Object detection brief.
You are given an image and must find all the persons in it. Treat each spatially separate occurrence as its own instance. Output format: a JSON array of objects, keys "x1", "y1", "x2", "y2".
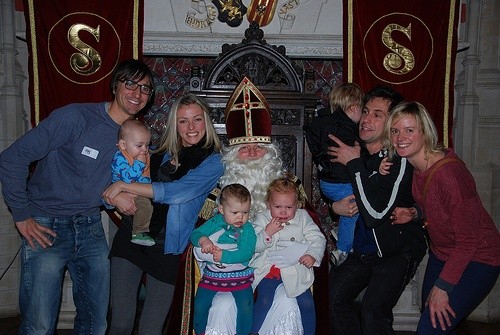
[
  {"x1": 101, "y1": 92, "x2": 226, "y2": 334},
  {"x1": 192, "y1": 125, "x2": 328, "y2": 335},
  {"x1": 384, "y1": 99, "x2": 500, "y2": 335},
  {"x1": 0, "y1": 59, "x2": 156, "y2": 335},
  {"x1": 327, "y1": 86, "x2": 430, "y2": 335},
  {"x1": 303, "y1": 83, "x2": 395, "y2": 267}
]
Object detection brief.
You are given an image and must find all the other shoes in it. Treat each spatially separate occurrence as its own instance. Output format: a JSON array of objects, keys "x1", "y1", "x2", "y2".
[{"x1": 131, "y1": 233, "x2": 156, "y2": 246}]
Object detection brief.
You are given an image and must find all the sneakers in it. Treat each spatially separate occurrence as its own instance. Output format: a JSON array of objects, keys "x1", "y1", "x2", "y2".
[
  {"x1": 331, "y1": 227, "x2": 353, "y2": 252},
  {"x1": 330, "y1": 249, "x2": 348, "y2": 267}
]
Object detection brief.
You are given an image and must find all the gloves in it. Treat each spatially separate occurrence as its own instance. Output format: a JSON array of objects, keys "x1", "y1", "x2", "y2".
[
  {"x1": 265, "y1": 240, "x2": 309, "y2": 269},
  {"x1": 195, "y1": 229, "x2": 239, "y2": 268}
]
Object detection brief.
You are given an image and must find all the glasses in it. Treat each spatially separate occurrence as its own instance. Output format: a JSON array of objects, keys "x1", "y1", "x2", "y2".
[
  {"x1": 118, "y1": 79, "x2": 152, "y2": 95},
  {"x1": 240, "y1": 145, "x2": 264, "y2": 152}
]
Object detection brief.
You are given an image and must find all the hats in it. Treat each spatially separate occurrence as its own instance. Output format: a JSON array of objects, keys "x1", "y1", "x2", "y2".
[{"x1": 224, "y1": 76, "x2": 272, "y2": 146}]
